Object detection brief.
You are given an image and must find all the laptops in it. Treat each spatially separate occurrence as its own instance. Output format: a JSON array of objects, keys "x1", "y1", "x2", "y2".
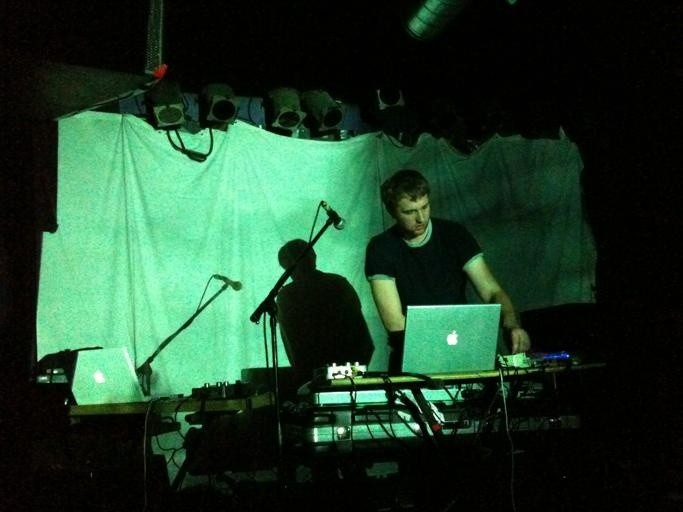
[
  {"x1": 58, "y1": 346, "x2": 156, "y2": 407},
  {"x1": 398, "y1": 302, "x2": 503, "y2": 375}
]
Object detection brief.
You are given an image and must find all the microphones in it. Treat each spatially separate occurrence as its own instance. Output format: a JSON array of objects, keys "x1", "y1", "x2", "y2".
[{"x1": 320, "y1": 199, "x2": 346, "y2": 231}]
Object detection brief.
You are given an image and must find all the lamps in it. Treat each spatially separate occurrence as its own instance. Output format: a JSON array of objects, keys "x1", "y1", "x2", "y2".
[
  {"x1": 301, "y1": 86, "x2": 347, "y2": 134},
  {"x1": 268, "y1": 85, "x2": 307, "y2": 134},
  {"x1": 149, "y1": 79, "x2": 186, "y2": 131},
  {"x1": 200, "y1": 79, "x2": 244, "y2": 125}
]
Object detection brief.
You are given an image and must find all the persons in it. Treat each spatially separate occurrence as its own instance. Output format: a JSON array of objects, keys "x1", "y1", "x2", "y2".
[
  {"x1": 365, "y1": 169, "x2": 531, "y2": 375},
  {"x1": 275, "y1": 239, "x2": 375, "y2": 367}
]
